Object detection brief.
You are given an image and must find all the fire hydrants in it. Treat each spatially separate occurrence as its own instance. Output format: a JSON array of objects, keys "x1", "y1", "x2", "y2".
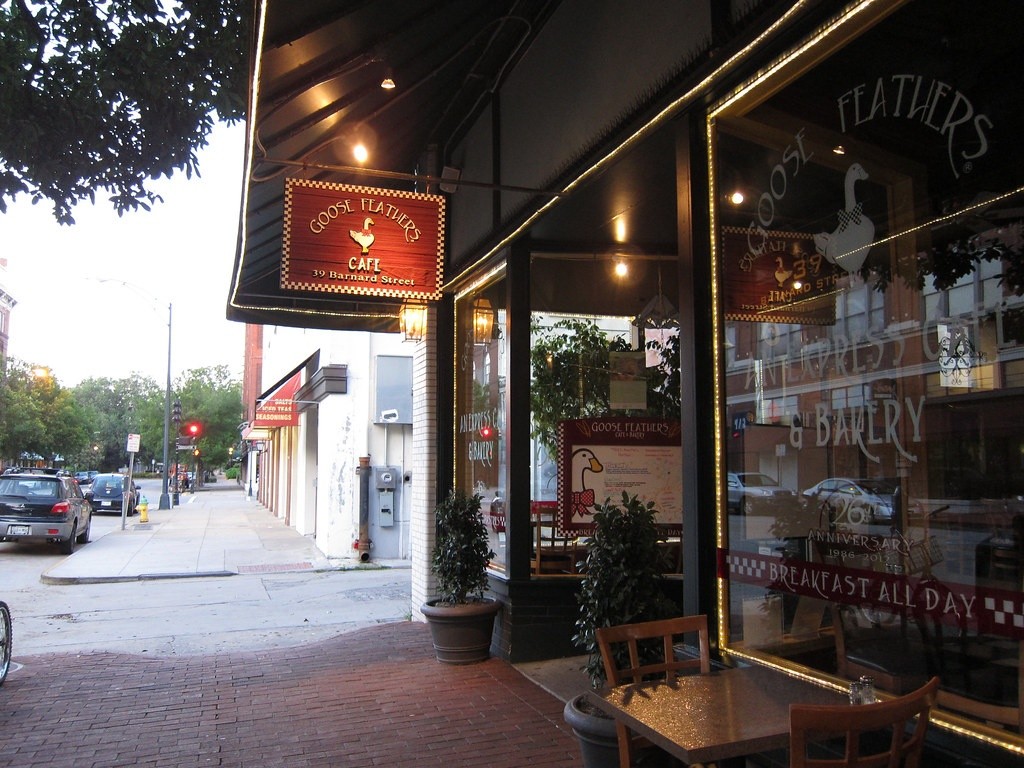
[{"x1": 136, "y1": 495, "x2": 150, "y2": 522}]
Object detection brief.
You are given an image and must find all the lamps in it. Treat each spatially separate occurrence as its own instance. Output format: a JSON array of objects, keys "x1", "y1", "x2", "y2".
[
  {"x1": 472, "y1": 293, "x2": 495, "y2": 347},
  {"x1": 631, "y1": 265, "x2": 679, "y2": 330},
  {"x1": 256, "y1": 440, "x2": 268, "y2": 457},
  {"x1": 398, "y1": 298, "x2": 428, "y2": 343}
]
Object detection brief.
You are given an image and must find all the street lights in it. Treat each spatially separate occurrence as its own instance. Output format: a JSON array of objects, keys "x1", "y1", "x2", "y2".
[
  {"x1": 99, "y1": 277, "x2": 172, "y2": 510},
  {"x1": 32, "y1": 367, "x2": 48, "y2": 378}
]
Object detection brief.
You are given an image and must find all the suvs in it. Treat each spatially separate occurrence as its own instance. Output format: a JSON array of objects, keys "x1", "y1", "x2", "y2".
[
  {"x1": 0, "y1": 466, "x2": 95, "y2": 555},
  {"x1": 727, "y1": 471, "x2": 796, "y2": 516},
  {"x1": 170, "y1": 472, "x2": 190, "y2": 490}
]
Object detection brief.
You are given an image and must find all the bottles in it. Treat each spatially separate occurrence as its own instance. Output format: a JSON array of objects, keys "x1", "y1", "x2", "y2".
[{"x1": 850, "y1": 675, "x2": 876, "y2": 705}]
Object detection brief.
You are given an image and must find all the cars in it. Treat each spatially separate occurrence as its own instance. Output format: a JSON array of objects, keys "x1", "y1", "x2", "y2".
[
  {"x1": 74, "y1": 471, "x2": 92, "y2": 484},
  {"x1": 90, "y1": 470, "x2": 100, "y2": 479},
  {"x1": 186, "y1": 472, "x2": 197, "y2": 483},
  {"x1": 84, "y1": 473, "x2": 141, "y2": 517},
  {"x1": 803, "y1": 477, "x2": 924, "y2": 527},
  {"x1": 3, "y1": 467, "x2": 72, "y2": 477}
]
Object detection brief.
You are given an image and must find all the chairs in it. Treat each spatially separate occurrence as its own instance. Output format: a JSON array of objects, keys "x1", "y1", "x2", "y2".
[
  {"x1": 594, "y1": 614, "x2": 747, "y2": 768},
  {"x1": 789, "y1": 675, "x2": 938, "y2": 767},
  {"x1": 530, "y1": 499, "x2": 578, "y2": 577}
]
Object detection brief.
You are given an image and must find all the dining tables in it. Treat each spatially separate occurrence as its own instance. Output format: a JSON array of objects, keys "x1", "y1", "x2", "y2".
[{"x1": 588, "y1": 666, "x2": 852, "y2": 768}]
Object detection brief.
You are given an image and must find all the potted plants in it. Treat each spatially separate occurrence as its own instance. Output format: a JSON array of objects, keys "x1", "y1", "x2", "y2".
[
  {"x1": 421, "y1": 485, "x2": 502, "y2": 665},
  {"x1": 562, "y1": 486, "x2": 683, "y2": 768}
]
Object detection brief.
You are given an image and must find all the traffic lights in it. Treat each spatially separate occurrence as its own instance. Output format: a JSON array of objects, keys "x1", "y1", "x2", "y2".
[{"x1": 190, "y1": 422, "x2": 200, "y2": 435}]
